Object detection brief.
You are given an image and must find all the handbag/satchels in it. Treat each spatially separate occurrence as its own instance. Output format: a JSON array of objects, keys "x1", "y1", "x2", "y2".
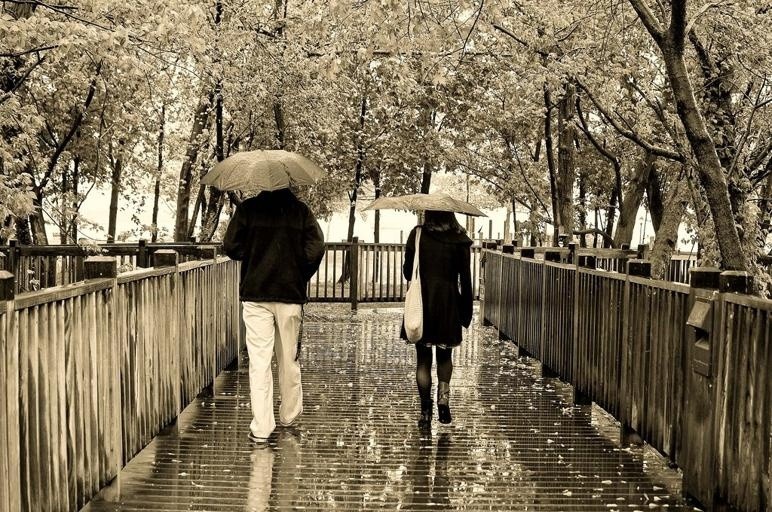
[{"x1": 402, "y1": 226, "x2": 427, "y2": 345}]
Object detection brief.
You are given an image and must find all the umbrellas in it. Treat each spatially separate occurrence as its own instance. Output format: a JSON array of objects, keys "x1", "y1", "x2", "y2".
[
  {"x1": 198, "y1": 147, "x2": 328, "y2": 192},
  {"x1": 361, "y1": 190, "x2": 488, "y2": 218}
]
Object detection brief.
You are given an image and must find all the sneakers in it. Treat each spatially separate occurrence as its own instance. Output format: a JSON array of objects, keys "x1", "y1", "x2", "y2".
[
  {"x1": 247, "y1": 430, "x2": 268, "y2": 444},
  {"x1": 279, "y1": 405, "x2": 304, "y2": 427}
]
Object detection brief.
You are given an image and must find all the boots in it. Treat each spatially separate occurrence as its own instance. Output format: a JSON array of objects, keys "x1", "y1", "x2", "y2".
[
  {"x1": 417, "y1": 398, "x2": 436, "y2": 430},
  {"x1": 438, "y1": 381, "x2": 452, "y2": 424}
]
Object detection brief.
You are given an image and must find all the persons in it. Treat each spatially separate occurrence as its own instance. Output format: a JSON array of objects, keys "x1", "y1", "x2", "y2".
[
  {"x1": 223, "y1": 187, "x2": 326, "y2": 445},
  {"x1": 244, "y1": 428, "x2": 301, "y2": 512},
  {"x1": 401, "y1": 421, "x2": 457, "y2": 511},
  {"x1": 400, "y1": 210, "x2": 473, "y2": 427}
]
